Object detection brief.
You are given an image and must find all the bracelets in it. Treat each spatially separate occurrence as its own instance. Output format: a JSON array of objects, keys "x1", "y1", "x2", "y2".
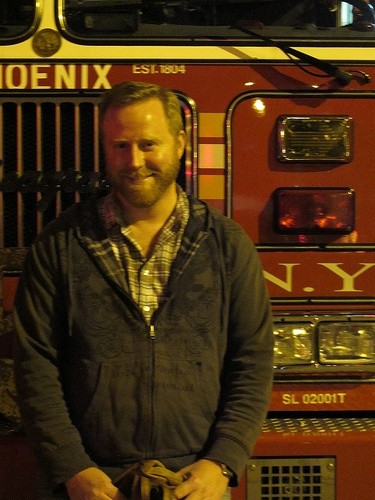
[{"x1": 204, "y1": 448, "x2": 241, "y2": 486}]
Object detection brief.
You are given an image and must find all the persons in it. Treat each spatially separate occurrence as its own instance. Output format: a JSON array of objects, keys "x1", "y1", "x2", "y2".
[{"x1": 11, "y1": 81, "x2": 275, "y2": 500}]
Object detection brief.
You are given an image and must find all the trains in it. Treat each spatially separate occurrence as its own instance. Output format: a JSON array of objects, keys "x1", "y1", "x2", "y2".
[{"x1": 0, "y1": 0, "x2": 375, "y2": 500}]
